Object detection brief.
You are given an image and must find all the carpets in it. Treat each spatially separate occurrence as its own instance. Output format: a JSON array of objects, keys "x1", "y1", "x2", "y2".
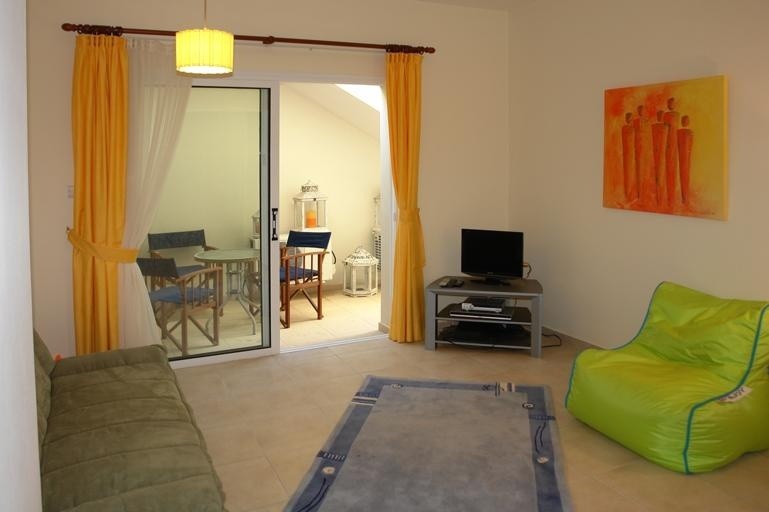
[{"x1": 277, "y1": 372, "x2": 576, "y2": 511}]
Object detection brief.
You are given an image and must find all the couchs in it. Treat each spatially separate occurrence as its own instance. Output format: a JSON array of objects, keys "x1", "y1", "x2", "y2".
[{"x1": 34, "y1": 330, "x2": 225, "y2": 511}]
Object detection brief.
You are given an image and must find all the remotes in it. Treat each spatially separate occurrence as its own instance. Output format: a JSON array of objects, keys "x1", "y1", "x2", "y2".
[
  {"x1": 455, "y1": 280, "x2": 464, "y2": 287},
  {"x1": 447, "y1": 279, "x2": 456, "y2": 288},
  {"x1": 439, "y1": 277, "x2": 450, "y2": 287}
]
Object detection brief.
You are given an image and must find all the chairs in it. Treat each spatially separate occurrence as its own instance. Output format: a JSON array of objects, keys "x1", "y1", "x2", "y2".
[
  {"x1": 132, "y1": 253, "x2": 225, "y2": 358},
  {"x1": 562, "y1": 277, "x2": 768, "y2": 478},
  {"x1": 247, "y1": 230, "x2": 334, "y2": 328},
  {"x1": 146, "y1": 228, "x2": 226, "y2": 316}
]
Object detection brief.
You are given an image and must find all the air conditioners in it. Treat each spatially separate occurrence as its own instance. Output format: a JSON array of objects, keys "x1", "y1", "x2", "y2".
[
  {"x1": 369, "y1": 226, "x2": 383, "y2": 282},
  {"x1": 250, "y1": 228, "x2": 334, "y2": 286}
]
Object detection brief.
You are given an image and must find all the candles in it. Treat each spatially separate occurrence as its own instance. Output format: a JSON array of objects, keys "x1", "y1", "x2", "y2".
[{"x1": 306, "y1": 210, "x2": 317, "y2": 229}]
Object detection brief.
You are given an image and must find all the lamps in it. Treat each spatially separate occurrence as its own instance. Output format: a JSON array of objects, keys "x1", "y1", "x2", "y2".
[{"x1": 171, "y1": 1, "x2": 236, "y2": 77}]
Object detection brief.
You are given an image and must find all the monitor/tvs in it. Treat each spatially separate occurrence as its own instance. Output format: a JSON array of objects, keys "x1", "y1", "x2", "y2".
[{"x1": 460, "y1": 228, "x2": 524, "y2": 285}]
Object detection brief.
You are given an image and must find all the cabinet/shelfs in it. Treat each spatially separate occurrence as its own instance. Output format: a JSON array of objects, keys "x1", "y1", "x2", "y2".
[{"x1": 423, "y1": 274, "x2": 546, "y2": 361}]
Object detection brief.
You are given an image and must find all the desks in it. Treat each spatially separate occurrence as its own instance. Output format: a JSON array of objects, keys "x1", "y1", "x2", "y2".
[{"x1": 192, "y1": 246, "x2": 258, "y2": 338}]
